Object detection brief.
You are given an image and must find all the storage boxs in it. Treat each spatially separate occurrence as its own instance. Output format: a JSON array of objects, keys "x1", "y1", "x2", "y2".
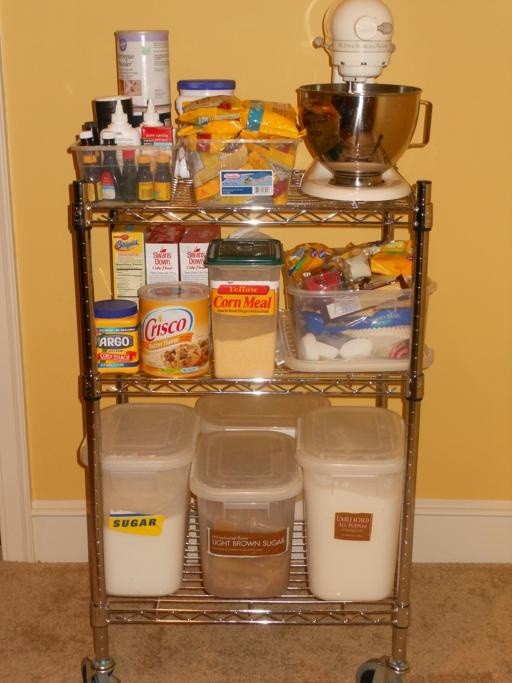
[
  {"x1": 205, "y1": 237, "x2": 438, "y2": 379},
  {"x1": 69, "y1": 140, "x2": 301, "y2": 208}
]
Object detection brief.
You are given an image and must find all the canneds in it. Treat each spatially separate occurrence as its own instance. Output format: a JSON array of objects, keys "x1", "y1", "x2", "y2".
[{"x1": 97, "y1": 299, "x2": 139, "y2": 374}]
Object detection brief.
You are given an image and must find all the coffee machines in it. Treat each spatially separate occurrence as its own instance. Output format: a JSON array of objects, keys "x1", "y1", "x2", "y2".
[{"x1": 294, "y1": 0, "x2": 433, "y2": 202}]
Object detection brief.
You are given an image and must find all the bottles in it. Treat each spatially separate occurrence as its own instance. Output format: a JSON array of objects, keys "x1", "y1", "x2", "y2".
[{"x1": 77, "y1": 96, "x2": 172, "y2": 204}]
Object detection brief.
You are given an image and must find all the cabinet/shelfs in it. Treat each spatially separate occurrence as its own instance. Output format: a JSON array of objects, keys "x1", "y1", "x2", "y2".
[{"x1": 73, "y1": 179, "x2": 432, "y2": 683}]
